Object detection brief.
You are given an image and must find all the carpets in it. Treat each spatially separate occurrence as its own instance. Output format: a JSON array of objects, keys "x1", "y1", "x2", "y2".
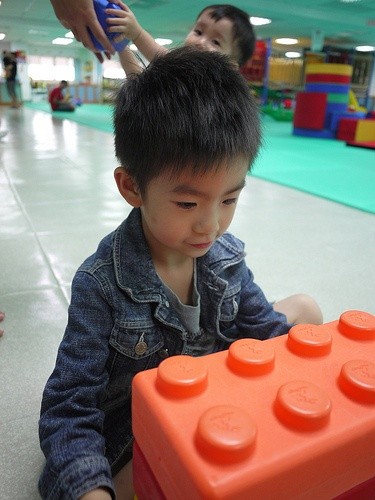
[{"x1": 23, "y1": 97, "x2": 375, "y2": 219}]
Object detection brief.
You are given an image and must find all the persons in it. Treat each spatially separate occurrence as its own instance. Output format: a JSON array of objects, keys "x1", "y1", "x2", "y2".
[
  {"x1": 2, "y1": 51, "x2": 20, "y2": 109},
  {"x1": 50, "y1": 0, "x2": 117, "y2": 62},
  {"x1": 103, "y1": 0, "x2": 256, "y2": 83},
  {"x1": 48, "y1": 79, "x2": 75, "y2": 111},
  {"x1": 33, "y1": 44, "x2": 322, "y2": 500}
]
